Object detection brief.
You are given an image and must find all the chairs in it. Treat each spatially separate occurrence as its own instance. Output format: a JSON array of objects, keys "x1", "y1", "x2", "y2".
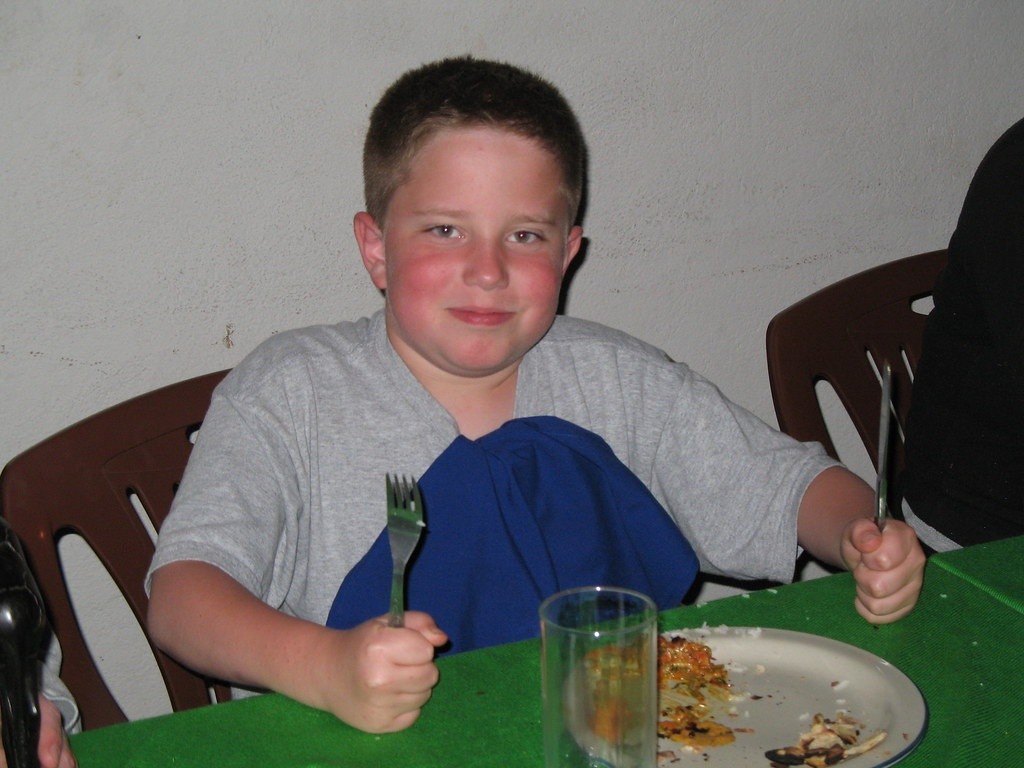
[
  {"x1": 767, "y1": 249, "x2": 969, "y2": 582},
  {"x1": 0, "y1": 368, "x2": 232, "y2": 731}
]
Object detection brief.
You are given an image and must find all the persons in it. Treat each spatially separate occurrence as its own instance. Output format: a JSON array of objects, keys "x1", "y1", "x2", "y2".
[
  {"x1": 900, "y1": 117, "x2": 1024, "y2": 559},
  {"x1": 0, "y1": 518, "x2": 82, "y2": 768},
  {"x1": 144, "y1": 55, "x2": 925, "y2": 735}
]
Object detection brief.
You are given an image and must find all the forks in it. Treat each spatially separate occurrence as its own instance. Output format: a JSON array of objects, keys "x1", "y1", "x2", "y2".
[{"x1": 385, "y1": 472, "x2": 423, "y2": 626}]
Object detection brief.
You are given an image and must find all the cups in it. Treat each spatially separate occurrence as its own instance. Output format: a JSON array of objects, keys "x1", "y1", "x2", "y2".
[{"x1": 538, "y1": 586, "x2": 659, "y2": 768}]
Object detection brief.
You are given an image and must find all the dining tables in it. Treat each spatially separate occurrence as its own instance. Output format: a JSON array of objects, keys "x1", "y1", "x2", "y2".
[{"x1": 71, "y1": 534, "x2": 1024, "y2": 768}]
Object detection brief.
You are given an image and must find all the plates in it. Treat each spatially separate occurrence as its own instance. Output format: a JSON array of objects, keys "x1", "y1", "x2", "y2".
[{"x1": 562, "y1": 626, "x2": 930, "y2": 768}]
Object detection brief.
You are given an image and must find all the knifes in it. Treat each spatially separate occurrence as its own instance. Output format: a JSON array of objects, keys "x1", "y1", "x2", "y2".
[{"x1": 873, "y1": 360, "x2": 892, "y2": 631}]
